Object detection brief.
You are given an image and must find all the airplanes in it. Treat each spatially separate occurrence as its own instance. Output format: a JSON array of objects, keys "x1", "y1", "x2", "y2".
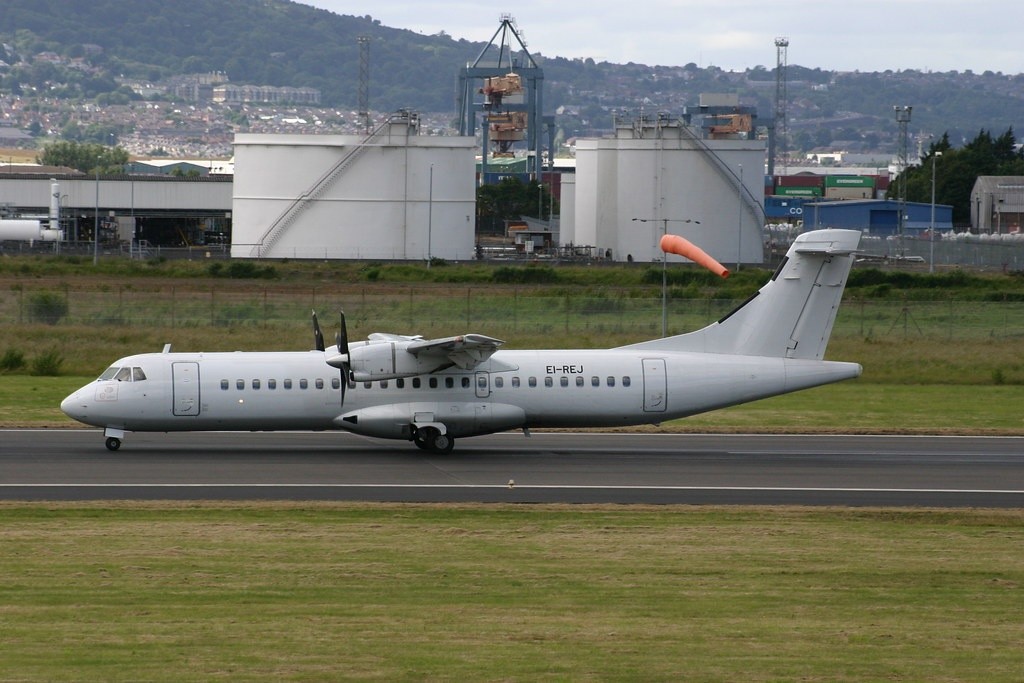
[{"x1": 60, "y1": 225, "x2": 864, "y2": 455}]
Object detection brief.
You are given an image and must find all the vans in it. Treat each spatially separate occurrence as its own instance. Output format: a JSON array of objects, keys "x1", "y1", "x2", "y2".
[{"x1": 203, "y1": 230, "x2": 231, "y2": 251}]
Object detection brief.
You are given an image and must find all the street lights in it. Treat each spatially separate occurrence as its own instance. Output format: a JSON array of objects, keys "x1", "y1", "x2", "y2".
[
  {"x1": 924, "y1": 150, "x2": 943, "y2": 274},
  {"x1": 94, "y1": 155, "x2": 103, "y2": 266},
  {"x1": 738, "y1": 161, "x2": 744, "y2": 279},
  {"x1": 127, "y1": 160, "x2": 135, "y2": 261},
  {"x1": 547, "y1": 160, "x2": 555, "y2": 231},
  {"x1": 537, "y1": 183, "x2": 542, "y2": 221},
  {"x1": 998, "y1": 198, "x2": 1004, "y2": 237}
]
